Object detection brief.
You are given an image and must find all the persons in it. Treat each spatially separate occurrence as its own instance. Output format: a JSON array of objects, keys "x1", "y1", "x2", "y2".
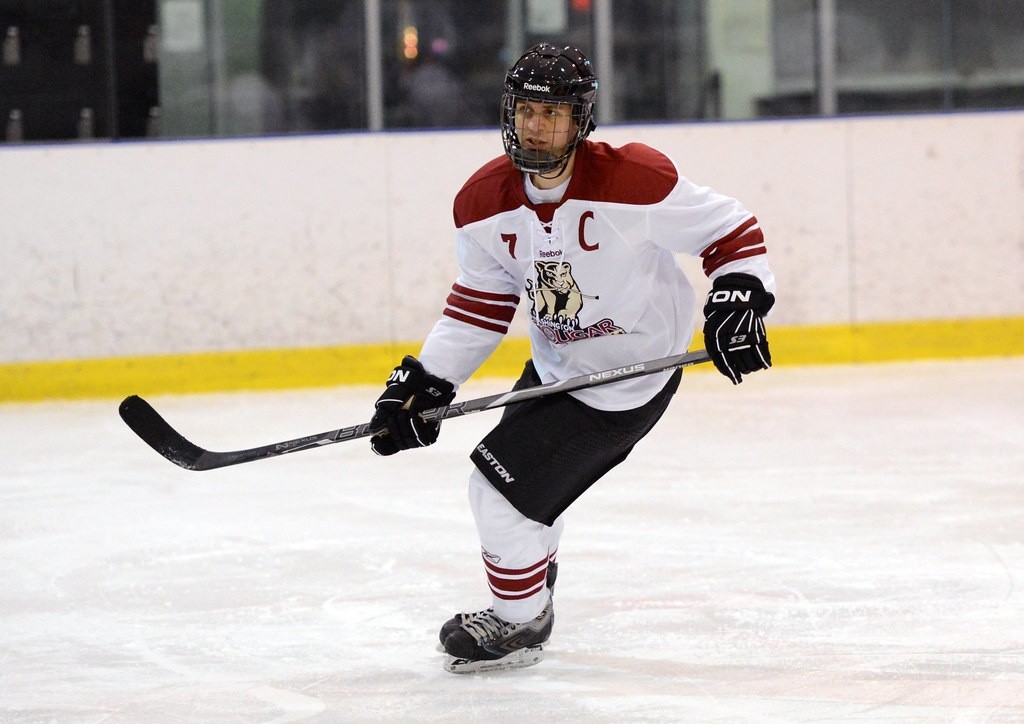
[
  {"x1": 370, "y1": 42, "x2": 776, "y2": 673},
  {"x1": 228, "y1": 31, "x2": 355, "y2": 135}
]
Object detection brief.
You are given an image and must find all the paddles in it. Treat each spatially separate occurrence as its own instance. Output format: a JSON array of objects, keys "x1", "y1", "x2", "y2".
[{"x1": 116, "y1": 349, "x2": 716, "y2": 472}]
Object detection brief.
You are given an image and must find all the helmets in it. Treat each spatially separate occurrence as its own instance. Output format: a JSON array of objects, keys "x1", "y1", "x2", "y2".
[{"x1": 500, "y1": 42, "x2": 599, "y2": 176}]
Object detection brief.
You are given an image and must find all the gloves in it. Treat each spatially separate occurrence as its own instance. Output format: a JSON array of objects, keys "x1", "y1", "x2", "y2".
[
  {"x1": 370, "y1": 356, "x2": 457, "y2": 456},
  {"x1": 703, "y1": 273, "x2": 774, "y2": 385}
]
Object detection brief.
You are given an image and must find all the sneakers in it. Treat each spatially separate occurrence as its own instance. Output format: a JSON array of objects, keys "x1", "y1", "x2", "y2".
[
  {"x1": 440, "y1": 561, "x2": 558, "y2": 640},
  {"x1": 444, "y1": 594, "x2": 555, "y2": 672}
]
